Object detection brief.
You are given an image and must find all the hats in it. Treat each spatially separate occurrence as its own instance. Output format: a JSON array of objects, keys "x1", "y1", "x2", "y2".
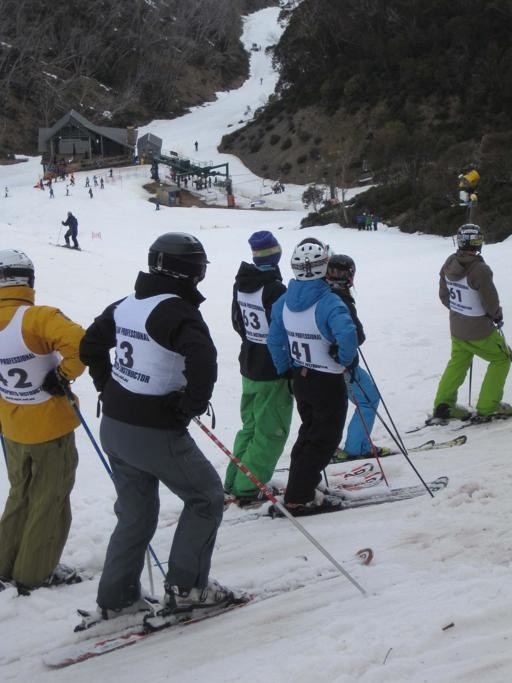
[{"x1": 248, "y1": 229, "x2": 282, "y2": 267}]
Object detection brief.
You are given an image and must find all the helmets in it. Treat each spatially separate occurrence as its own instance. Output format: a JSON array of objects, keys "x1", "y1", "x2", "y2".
[
  {"x1": 147, "y1": 230, "x2": 211, "y2": 286},
  {"x1": 0, "y1": 248, "x2": 35, "y2": 290},
  {"x1": 325, "y1": 253, "x2": 357, "y2": 288},
  {"x1": 290, "y1": 236, "x2": 331, "y2": 282},
  {"x1": 455, "y1": 222, "x2": 484, "y2": 253}
]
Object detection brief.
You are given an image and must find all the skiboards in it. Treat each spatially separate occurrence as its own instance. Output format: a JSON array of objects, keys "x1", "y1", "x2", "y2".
[
  {"x1": 221, "y1": 437, "x2": 467, "y2": 518},
  {"x1": 41, "y1": 549, "x2": 374, "y2": 670},
  {"x1": 405, "y1": 412, "x2": 509, "y2": 432}
]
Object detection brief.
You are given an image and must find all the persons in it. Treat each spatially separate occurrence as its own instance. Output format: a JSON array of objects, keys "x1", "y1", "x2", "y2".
[
  {"x1": 194, "y1": 139, "x2": 199, "y2": 151},
  {"x1": 223, "y1": 229, "x2": 297, "y2": 508},
  {"x1": 75, "y1": 230, "x2": 239, "y2": 623},
  {"x1": 434, "y1": 221, "x2": 511, "y2": 418},
  {"x1": 355, "y1": 211, "x2": 379, "y2": 230},
  {"x1": 155, "y1": 193, "x2": 161, "y2": 211},
  {"x1": 263, "y1": 238, "x2": 359, "y2": 519},
  {"x1": 5, "y1": 186, "x2": 10, "y2": 196},
  {"x1": 322, "y1": 253, "x2": 391, "y2": 459},
  {"x1": 1, "y1": 245, "x2": 90, "y2": 597},
  {"x1": 169, "y1": 163, "x2": 219, "y2": 189},
  {"x1": 61, "y1": 212, "x2": 81, "y2": 248},
  {"x1": 271, "y1": 181, "x2": 285, "y2": 193},
  {"x1": 36, "y1": 157, "x2": 116, "y2": 198}
]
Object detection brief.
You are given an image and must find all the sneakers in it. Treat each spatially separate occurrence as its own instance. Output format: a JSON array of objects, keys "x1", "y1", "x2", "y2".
[
  {"x1": 15, "y1": 563, "x2": 83, "y2": 592},
  {"x1": 223, "y1": 483, "x2": 281, "y2": 506},
  {"x1": 433, "y1": 402, "x2": 512, "y2": 421},
  {"x1": 343, "y1": 444, "x2": 384, "y2": 459},
  {"x1": 62, "y1": 242, "x2": 79, "y2": 249},
  {"x1": 162, "y1": 579, "x2": 236, "y2": 616},
  {"x1": 282, "y1": 485, "x2": 352, "y2": 514},
  {"x1": 94, "y1": 590, "x2": 162, "y2": 621}
]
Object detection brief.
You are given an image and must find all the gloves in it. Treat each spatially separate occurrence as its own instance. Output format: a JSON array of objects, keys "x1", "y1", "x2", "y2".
[
  {"x1": 491, "y1": 306, "x2": 504, "y2": 325},
  {"x1": 281, "y1": 366, "x2": 295, "y2": 381},
  {"x1": 329, "y1": 342, "x2": 360, "y2": 370},
  {"x1": 172, "y1": 385, "x2": 210, "y2": 427},
  {"x1": 41, "y1": 366, "x2": 70, "y2": 399}
]
